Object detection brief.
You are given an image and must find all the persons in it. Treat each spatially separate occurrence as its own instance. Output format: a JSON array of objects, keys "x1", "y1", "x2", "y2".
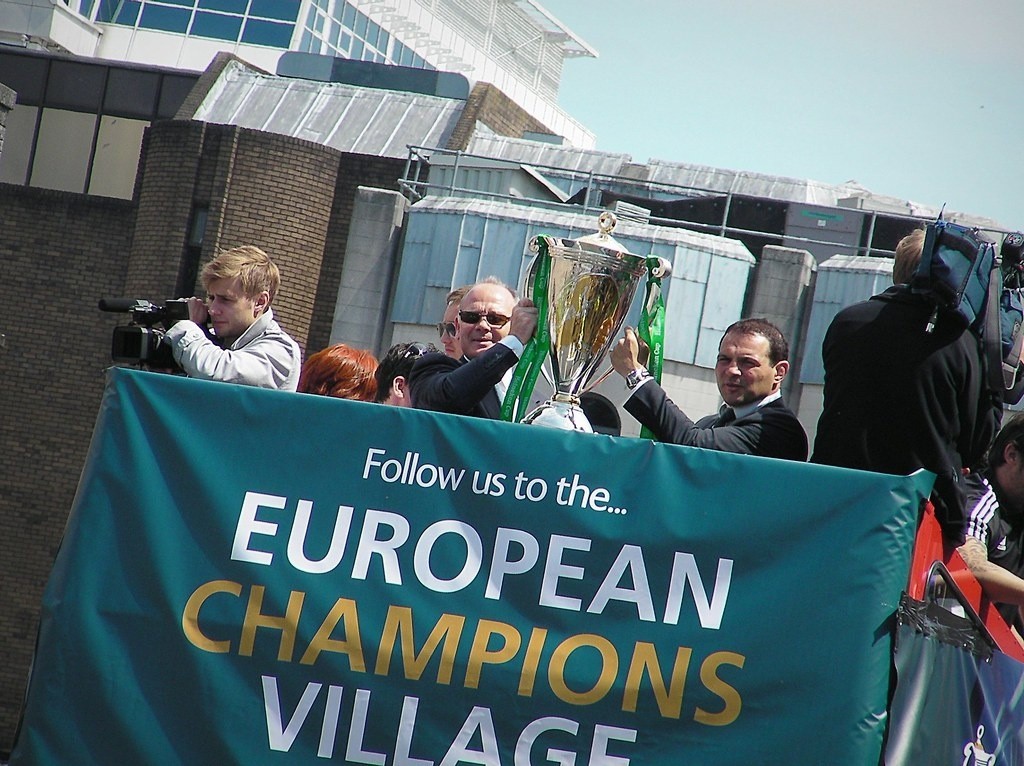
[
  {"x1": 437, "y1": 284, "x2": 474, "y2": 361},
  {"x1": 408, "y1": 276, "x2": 539, "y2": 420},
  {"x1": 811, "y1": 227, "x2": 1024, "y2": 640},
  {"x1": 375, "y1": 340, "x2": 443, "y2": 407},
  {"x1": 608, "y1": 317, "x2": 809, "y2": 462},
  {"x1": 163, "y1": 245, "x2": 302, "y2": 393},
  {"x1": 297, "y1": 344, "x2": 380, "y2": 404}
]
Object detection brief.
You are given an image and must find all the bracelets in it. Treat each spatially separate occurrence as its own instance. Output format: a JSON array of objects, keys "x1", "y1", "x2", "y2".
[{"x1": 626, "y1": 368, "x2": 650, "y2": 389}]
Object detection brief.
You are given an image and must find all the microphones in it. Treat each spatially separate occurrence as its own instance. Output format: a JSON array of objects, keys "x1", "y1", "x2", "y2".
[{"x1": 98, "y1": 299, "x2": 155, "y2": 313}]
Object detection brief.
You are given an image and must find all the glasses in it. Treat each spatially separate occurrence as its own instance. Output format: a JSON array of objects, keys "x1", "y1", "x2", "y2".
[
  {"x1": 388, "y1": 342, "x2": 428, "y2": 383},
  {"x1": 459, "y1": 311, "x2": 511, "y2": 329},
  {"x1": 436, "y1": 323, "x2": 457, "y2": 338}
]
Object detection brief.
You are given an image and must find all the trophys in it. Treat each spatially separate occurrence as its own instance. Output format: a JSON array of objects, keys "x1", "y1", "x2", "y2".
[{"x1": 517, "y1": 212, "x2": 672, "y2": 431}]
